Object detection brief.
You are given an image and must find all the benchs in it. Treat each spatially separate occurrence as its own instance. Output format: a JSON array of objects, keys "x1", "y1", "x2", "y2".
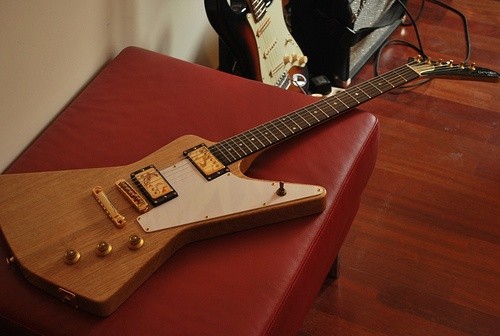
[{"x1": 1, "y1": 43, "x2": 380, "y2": 335}]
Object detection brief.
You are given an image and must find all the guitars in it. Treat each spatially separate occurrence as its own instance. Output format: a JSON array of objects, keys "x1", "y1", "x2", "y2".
[
  {"x1": 0, "y1": 55, "x2": 499, "y2": 317},
  {"x1": 204, "y1": 0, "x2": 308, "y2": 93}
]
